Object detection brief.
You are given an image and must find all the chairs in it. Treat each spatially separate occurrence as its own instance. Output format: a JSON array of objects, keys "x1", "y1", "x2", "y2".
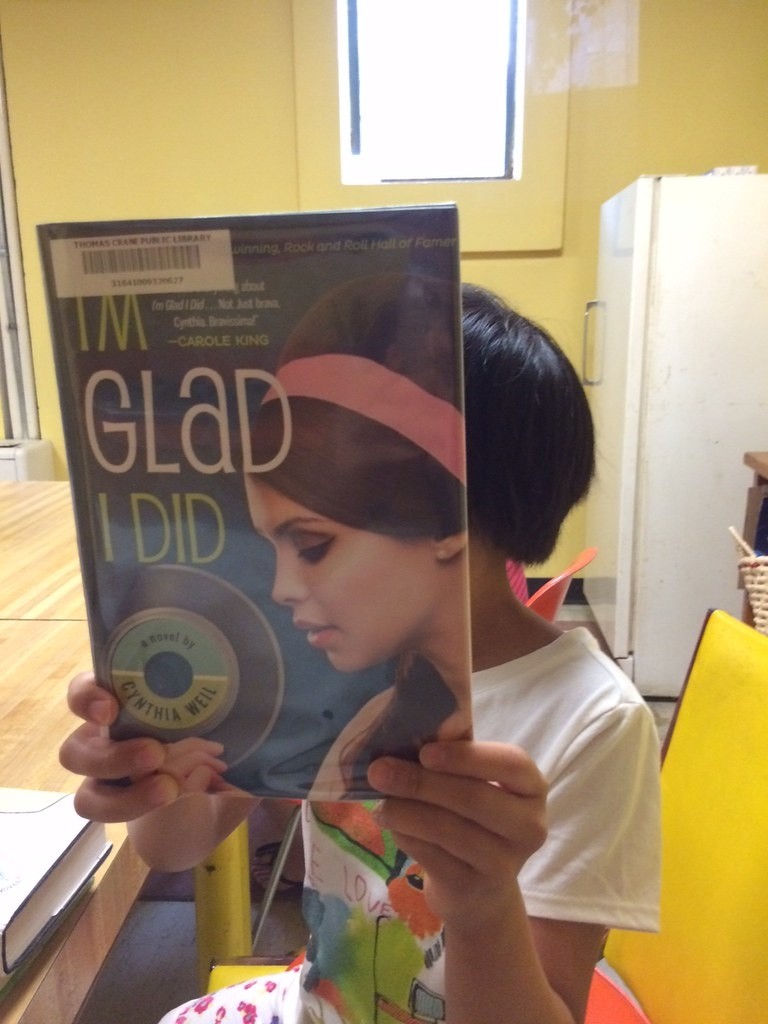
[{"x1": 206, "y1": 608, "x2": 768, "y2": 1024}]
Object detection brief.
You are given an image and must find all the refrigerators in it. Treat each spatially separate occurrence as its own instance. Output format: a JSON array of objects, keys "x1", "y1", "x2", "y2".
[{"x1": 581, "y1": 174, "x2": 768, "y2": 699}]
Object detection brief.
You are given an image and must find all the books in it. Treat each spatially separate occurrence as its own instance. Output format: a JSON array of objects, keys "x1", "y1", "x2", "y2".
[{"x1": 34, "y1": 204, "x2": 476, "y2": 798}]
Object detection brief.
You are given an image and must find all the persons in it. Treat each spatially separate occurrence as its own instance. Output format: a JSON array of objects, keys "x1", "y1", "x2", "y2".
[
  {"x1": 242, "y1": 269, "x2": 474, "y2": 799},
  {"x1": 59, "y1": 282, "x2": 663, "y2": 1024}
]
